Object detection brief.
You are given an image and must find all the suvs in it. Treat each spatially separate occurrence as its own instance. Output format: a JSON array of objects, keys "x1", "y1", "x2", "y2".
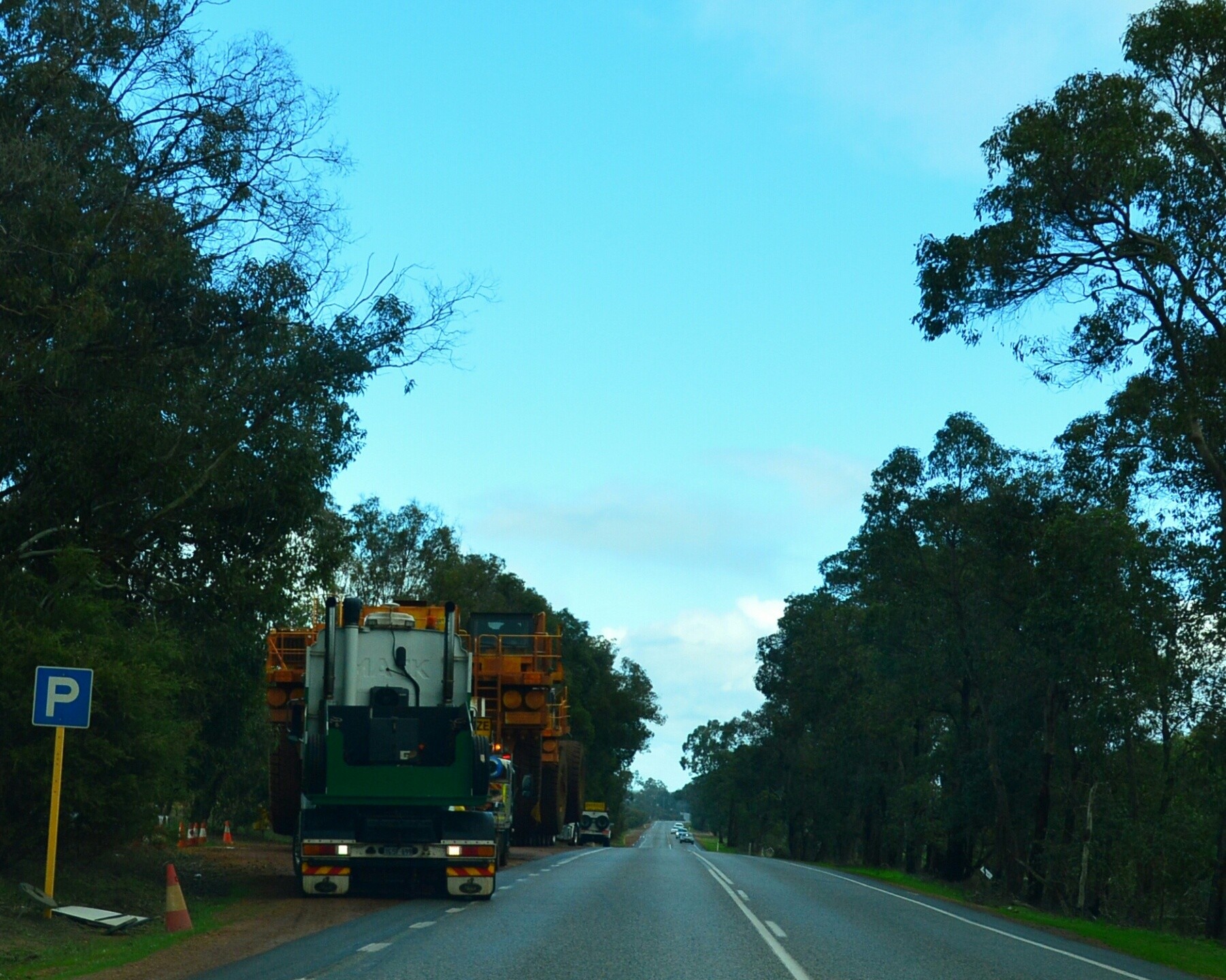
[{"x1": 576, "y1": 811, "x2": 615, "y2": 847}]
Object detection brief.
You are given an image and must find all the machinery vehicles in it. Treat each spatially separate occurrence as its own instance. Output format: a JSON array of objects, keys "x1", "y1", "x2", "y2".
[{"x1": 261, "y1": 590, "x2": 589, "y2": 903}]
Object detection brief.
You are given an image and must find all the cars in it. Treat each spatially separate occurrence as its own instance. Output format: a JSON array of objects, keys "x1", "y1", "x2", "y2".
[{"x1": 670, "y1": 822, "x2": 695, "y2": 845}]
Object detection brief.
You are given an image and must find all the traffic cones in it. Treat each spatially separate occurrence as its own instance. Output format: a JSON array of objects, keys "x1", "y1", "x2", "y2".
[
  {"x1": 197, "y1": 822, "x2": 208, "y2": 845},
  {"x1": 221, "y1": 820, "x2": 234, "y2": 847},
  {"x1": 192, "y1": 821, "x2": 199, "y2": 845},
  {"x1": 185, "y1": 822, "x2": 196, "y2": 846},
  {"x1": 163, "y1": 863, "x2": 193, "y2": 933},
  {"x1": 175, "y1": 821, "x2": 187, "y2": 848}
]
